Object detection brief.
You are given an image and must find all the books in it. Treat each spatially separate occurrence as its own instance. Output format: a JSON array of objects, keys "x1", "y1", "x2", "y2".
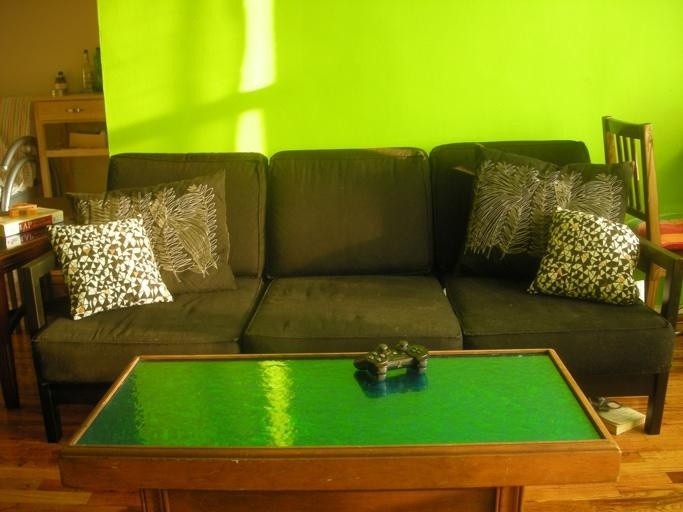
[
  {"x1": 0, "y1": 203, "x2": 64, "y2": 250},
  {"x1": 588, "y1": 396, "x2": 646, "y2": 436}
]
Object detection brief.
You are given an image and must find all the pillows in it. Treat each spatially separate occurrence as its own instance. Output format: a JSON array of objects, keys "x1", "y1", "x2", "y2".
[
  {"x1": 524, "y1": 204, "x2": 642, "y2": 306},
  {"x1": 40, "y1": 214, "x2": 174, "y2": 323},
  {"x1": 461, "y1": 143, "x2": 634, "y2": 283},
  {"x1": 66, "y1": 165, "x2": 239, "y2": 295}
]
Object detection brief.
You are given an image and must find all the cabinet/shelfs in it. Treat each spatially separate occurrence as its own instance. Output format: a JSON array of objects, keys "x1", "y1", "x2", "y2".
[{"x1": 32, "y1": 91, "x2": 108, "y2": 198}]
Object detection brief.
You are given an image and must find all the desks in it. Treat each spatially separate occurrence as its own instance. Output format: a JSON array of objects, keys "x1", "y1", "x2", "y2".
[
  {"x1": 0, "y1": 234, "x2": 56, "y2": 411},
  {"x1": 59, "y1": 346, "x2": 621, "y2": 512}
]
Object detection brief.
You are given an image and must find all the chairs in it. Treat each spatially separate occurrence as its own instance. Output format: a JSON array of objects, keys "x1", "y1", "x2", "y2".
[
  {"x1": 601, "y1": 114, "x2": 682, "y2": 334},
  {"x1": 1, "y1": 95, "x2": 61, "y2": 213}
]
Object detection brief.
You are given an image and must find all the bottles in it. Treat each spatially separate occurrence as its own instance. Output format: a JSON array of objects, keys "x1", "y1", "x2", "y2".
[
  {"x1": 82, "y1": 47, "x2": 102, "y2": 94},
  {"x1": 54, "y1": 71, "x2": 68, "y2": 92}
]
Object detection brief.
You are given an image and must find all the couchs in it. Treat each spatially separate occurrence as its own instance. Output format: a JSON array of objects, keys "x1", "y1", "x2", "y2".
[{"x1": 17, "y1": 139, "x2": 683, "y2": 444}]
